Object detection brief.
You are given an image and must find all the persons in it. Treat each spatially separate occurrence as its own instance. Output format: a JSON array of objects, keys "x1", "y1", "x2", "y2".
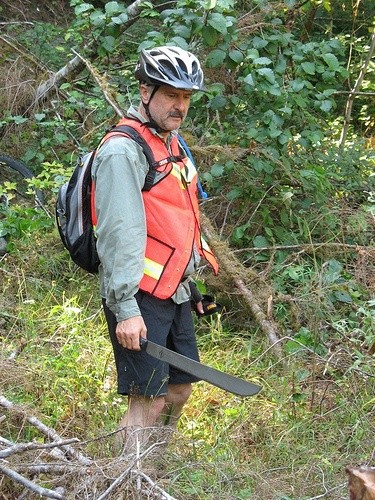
[{"x1": 88, "y1": 46, "x2": 219, "y2": 477}]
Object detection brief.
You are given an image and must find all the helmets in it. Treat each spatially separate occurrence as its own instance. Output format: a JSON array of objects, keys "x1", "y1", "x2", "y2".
[{"x1": 131, "y1": 45, "x2": 204, "y2": 91}]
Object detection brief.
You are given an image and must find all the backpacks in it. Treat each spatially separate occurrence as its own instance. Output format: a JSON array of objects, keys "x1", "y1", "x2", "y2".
[{"x1": 56, "y1": 125, "x2": 156, "y2": 275}]
